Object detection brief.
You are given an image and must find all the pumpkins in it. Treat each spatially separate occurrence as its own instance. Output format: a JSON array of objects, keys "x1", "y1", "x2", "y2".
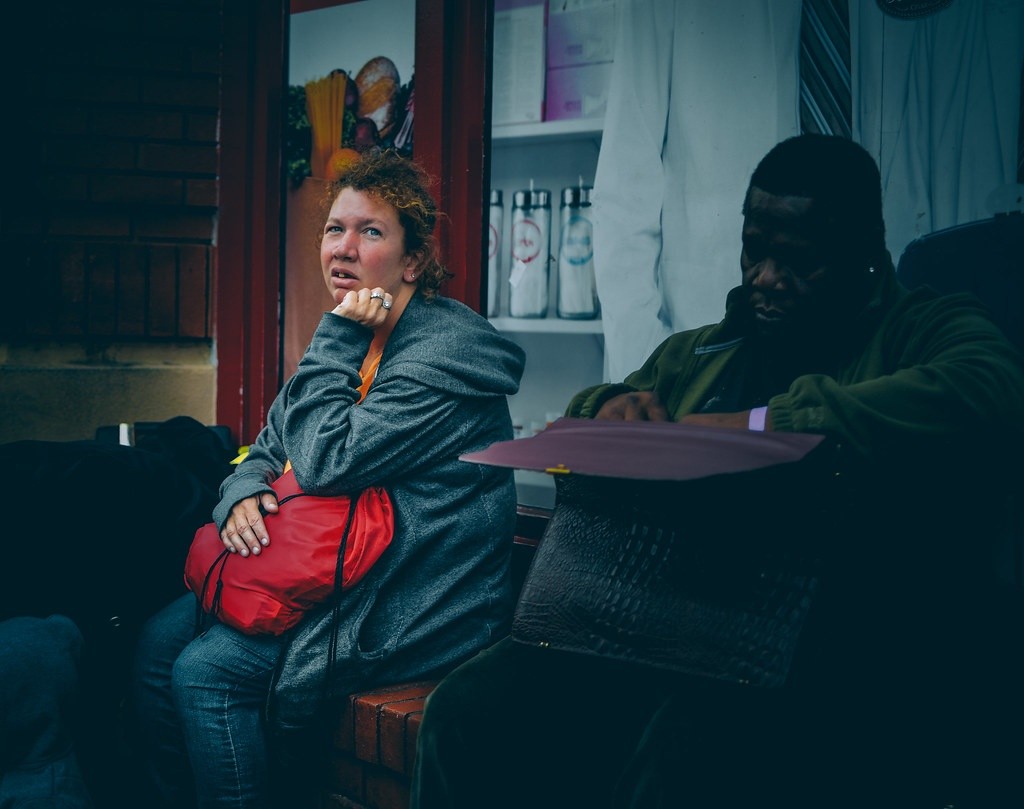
[{"x1": 326, "y1": 149, "x2": 359, "y2": 179}]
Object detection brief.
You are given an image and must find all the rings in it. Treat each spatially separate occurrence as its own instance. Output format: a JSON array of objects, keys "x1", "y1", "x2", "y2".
[
  {"x1": 382, "y1": 302, "x2": 392, "y2": 310},
  {"x1": 370, "y1": 294, "x2": 383, "y2": 302}
]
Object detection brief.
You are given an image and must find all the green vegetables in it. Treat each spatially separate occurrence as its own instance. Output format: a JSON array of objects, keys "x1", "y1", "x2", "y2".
[
  {"x1": 343, "y1": 112, "x2": 357, "y2": 147},
  {"x1": 287, "y1": 85, "x2": 311, "y2": 190}
]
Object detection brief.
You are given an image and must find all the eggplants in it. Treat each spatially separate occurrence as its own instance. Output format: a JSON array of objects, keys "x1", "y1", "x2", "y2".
[
  {"x1": 352, "y1": 118, "x2": 379, "y2": 151},
  {"x1": 329, "y1": 69, "x2": 358, "y2": 109},
  {"x1": 395, "y1": 74, "x2": 415, "y2": 161}
]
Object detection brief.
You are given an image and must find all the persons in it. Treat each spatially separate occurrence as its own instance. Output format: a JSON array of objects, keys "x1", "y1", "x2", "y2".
[
  {"x1": 410, "y1": 134, "x2": 1024, "y2": 809},
  {"x1": 130, "y1": 145, "x2": 527, "y2": 809},
  {"x1": 589, "y1": 0, "x2": 1024, "y2": 385}
]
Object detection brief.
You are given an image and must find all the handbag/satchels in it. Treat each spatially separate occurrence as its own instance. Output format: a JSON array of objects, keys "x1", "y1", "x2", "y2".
[
  {"x1": 184, "y1": 468, "x2": 394, "y2": 637},
  {"x1": 458, "y1": 417, "x2": 855, "y2": 699}
]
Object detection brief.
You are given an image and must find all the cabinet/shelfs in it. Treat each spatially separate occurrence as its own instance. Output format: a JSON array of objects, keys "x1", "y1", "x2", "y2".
[{"x1": 487, "y1": 115, "x2": 613, "y2": 515}]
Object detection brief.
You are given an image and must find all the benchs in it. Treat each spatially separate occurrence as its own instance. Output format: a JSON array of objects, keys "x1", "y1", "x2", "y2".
[{"x1": 316, "y1": 505, "x2": 559, "y2": 809}]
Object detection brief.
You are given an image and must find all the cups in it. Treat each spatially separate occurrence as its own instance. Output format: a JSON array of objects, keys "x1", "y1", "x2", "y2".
[
  {"x1": 556, "y1": 184, "x2": 599, "y2": 320},
  {"x1": 488, "y1": 189, "x2": 502, "y2": 318},
  {"x1": 507, "y1": 187, "x2": 552, "y2": 318}
]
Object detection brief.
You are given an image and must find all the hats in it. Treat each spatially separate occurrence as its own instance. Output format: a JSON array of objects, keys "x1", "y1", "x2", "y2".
[{"x1": 741, "y1": 133, "x2": 884, "y2": 240}]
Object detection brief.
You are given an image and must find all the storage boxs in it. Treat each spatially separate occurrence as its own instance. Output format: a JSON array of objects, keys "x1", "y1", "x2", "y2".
[{"x1": 491, "y1": 1, "x2": 619, "y2": 124}]
